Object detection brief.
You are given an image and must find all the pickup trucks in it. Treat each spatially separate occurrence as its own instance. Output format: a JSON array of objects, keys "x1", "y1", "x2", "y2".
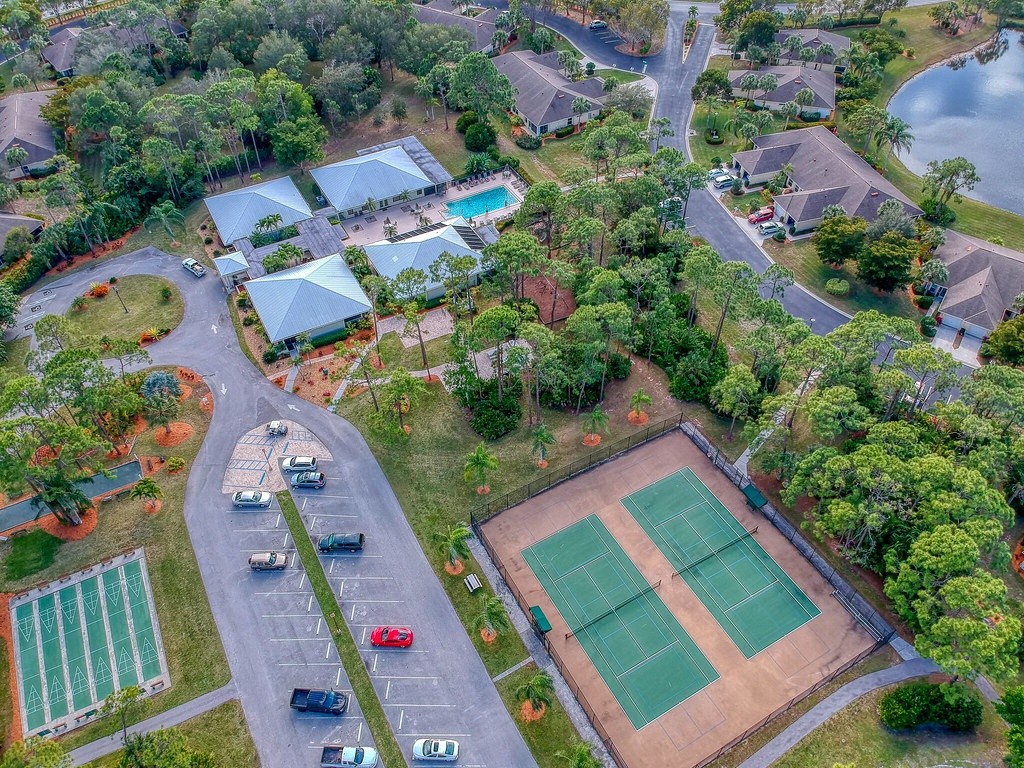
[
  {"x1": 289, "y1": 687, "x2": 349, "y2": 715},
  {"x1": 181, "y1": 257, "x2": 208, "y2": 277},
  {"x1": 321, "y1": 741, "x2": 379, "y2": 768}
]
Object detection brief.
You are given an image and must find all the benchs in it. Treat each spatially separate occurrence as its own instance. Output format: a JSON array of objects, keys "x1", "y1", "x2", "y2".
[
  {"x1": 115, "y1": 488, "x2": 131, "y2": 497},
  {"x1": 11, "y1": 528, "x2": 27, "y2": 536},
  {"x1": 464, "y1": 579, "x2": 473, "y2": 592},
  {"x1": 474, "y1": 574, "x2": 482, "y2": 587}
]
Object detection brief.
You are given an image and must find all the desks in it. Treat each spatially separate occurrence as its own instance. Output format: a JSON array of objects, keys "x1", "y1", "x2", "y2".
[
  {"x1": 467, "y1": 574, "x2": 479, "y2": 589},
  {"x1": 504, "y1": 171, "x2": 510, "y2": 177}
]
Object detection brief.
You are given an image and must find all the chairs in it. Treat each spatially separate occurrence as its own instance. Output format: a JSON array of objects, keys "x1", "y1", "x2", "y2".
[
  {"x1": 466, "y1": 178, "x2": 473, "y2": 187},
  {"x1": 522, "y1": 187, "x2": 529, "y2": 196},
  {"x1": 511, "y1": 176, "x2": 521, "y2": 184},
  {"x1": 483, "y1": 172, "x2": 489, "y2": 181},
  {"x1": 478, "y1": 174, "x2": 484, "y2": 184},
  {"x1": 520, "y1": 184, "x2": 527, "y2": 191},
  {"x1": 455, "y1": 180, "x2": 462, "y2": 191},
  {"x1": 472, "y1": 176, "x2": 478, "y2": 185},
  {"x1": 489, "y1": 169, "x2": 495, "y2": 179},
  {"x1": 514, "y1": 181, "x2": 523, "y2": 189}
]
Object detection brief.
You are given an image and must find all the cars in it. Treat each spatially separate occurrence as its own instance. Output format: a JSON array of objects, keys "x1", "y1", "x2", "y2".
[
  {"x1": 412, "y1": 737, "x2": 460, "y2": 763},
  {"x1": 319, "y1": 532, "x2": 364, "y2": 553},
  {"x1": 233, "y1": 490, "x2": 273, "y2": 508},
  {"x1": 709, "y1": 168, "x2": 729, "y2": 179},
  {"x1": 713, "y1": 175, "x2": 736, "y2": 188},
  {"x1": 748, "y1": 209, "x2": 774, "y2": 224},
  {"x1": 291, "y1": 472, "x2": 326, "y2": 489},
  {"x1": 269, "y1": 420, "x2": 288, "y2": 436},
  {"x1": 371, "y1": 625, "x2": 414, "y2": 648},
  {"x1": 282, "y1": 456, "x2": 318, "y2": 472},
  {"x1": 248, "y1": 551, "x2": 287, "y2": 571},
  {"x1": 658, "y1": 197, "x2": 683, "y2": 210},
  {"x1": 589, "y1": 19, "x2": 608, "y2": 29},
  {"x1": 758, "y1": 222, "x2": 784, "y2": 236}
]
